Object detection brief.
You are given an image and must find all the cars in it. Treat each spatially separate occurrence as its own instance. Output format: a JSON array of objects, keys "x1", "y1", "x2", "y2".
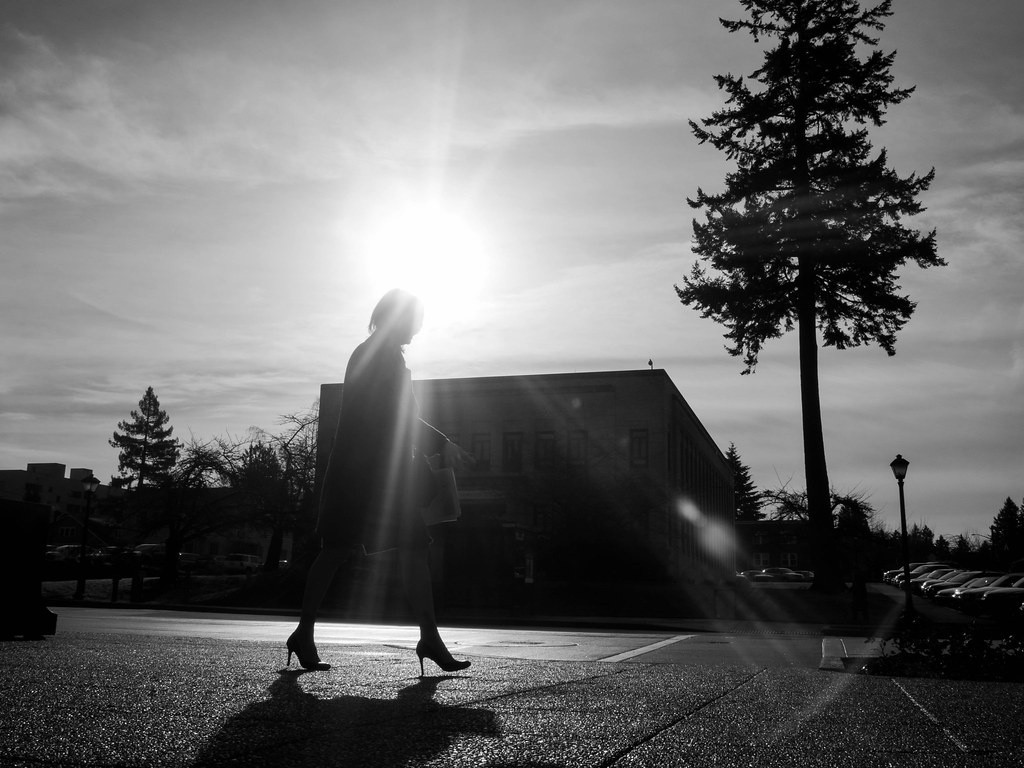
[
  {"x1": 981, "y1": 578, "x2": 1024, "y2": 614},
  {"x1": 736, "y1": 565, "x2": 814, "y2": 586},
  {"x1": 883, "y1": 561, "x2": 1024, "y2": 613},
  {"x1": 41, "y1": 542, "x2": 290, "y2": 574}
]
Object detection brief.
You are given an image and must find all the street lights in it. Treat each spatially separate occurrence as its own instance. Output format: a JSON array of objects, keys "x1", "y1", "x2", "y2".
[{"x1": 889, "y1": 454, "x2": 924, "y2": 634}]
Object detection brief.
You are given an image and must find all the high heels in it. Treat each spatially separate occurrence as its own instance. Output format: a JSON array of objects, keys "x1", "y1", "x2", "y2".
[
  {"x1": 286, "y1": 632, "x2": 330, "y2": 670},
  {"x1": 416, "y1": 640, "x2": 470, "y2": 676}
]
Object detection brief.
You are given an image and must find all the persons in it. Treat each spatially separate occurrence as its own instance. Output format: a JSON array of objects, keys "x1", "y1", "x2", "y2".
[{"x1": 280, "y1": 287, "x2": 473, "y2": 681}]
